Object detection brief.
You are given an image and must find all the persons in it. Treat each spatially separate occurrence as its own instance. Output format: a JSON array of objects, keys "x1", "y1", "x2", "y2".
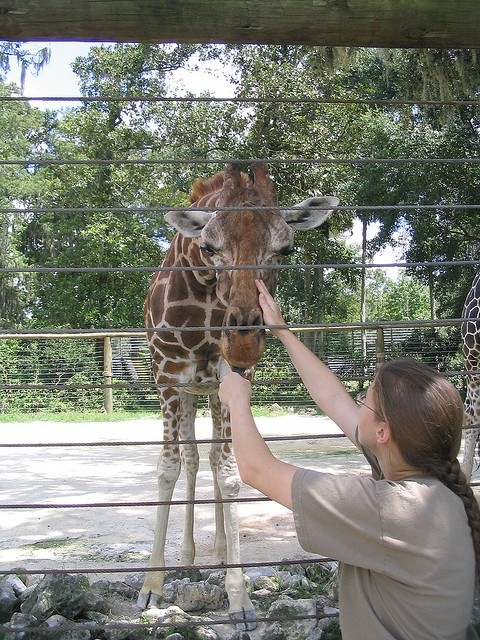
[{"x1": 217, "y1": 278, "x2": 479, "y2": 640}]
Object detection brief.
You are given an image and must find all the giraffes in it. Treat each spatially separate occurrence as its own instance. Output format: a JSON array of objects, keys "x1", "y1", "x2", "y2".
[
  {"x1": 460, "y1": 269, "x2": 480, "y2": 488},
  {"x1": 136, "y1": 162, "x2": 340, "y2": 632}
]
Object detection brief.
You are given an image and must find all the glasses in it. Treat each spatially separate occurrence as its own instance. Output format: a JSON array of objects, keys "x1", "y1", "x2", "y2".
[{"x1": 354, "y1": 389, "x2": 384, "y2": 421}]
